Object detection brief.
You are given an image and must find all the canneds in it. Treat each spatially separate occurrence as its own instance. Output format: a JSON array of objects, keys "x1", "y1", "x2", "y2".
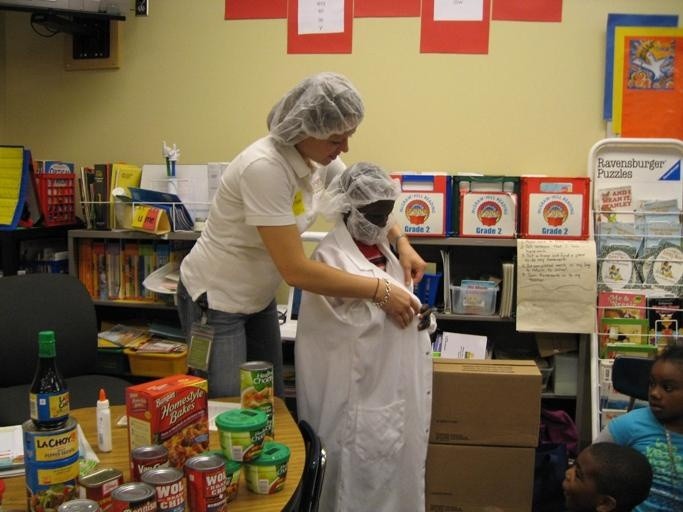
[
  {"x1": 239, "y1": 360, "x2": 275, "y2": 443},
  {"x1": 78, "y1": 467, "x2": 125, "y2": 512},
  {"x1": 58, "y1": 498, "x2": 102, "y2": 512},
  {"x1": 140, "y1": 467, "x2": 186, "y2": 512},
  {"x1": 110, "y1": 481, "x2": 158, "y2": 512},
  {"x1": 184, "y1": 455, "x2": 228, "y2": 512},
  {"x1": 129, "y1": 445, "x2": 170, "y2": 482},
  {"x1": 21, "y1": 415, "x2": 81, "y2": 511}
]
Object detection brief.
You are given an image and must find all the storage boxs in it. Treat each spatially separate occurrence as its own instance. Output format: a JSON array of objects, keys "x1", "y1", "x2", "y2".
[
  {"x1": 522, "y1": 176, "x2": 593, "y2": 239},
  {"x1": 27, "y1": 171, "x2": 79, "y2": 229},
  {"x1": 494, "y1": 346, "x2": 555, "y2": 394},
  {"x1": 122, "y1": 344, "x2": 190, "y2": 379},
  {"x1": 451, "y1": 174, "x2": 522, "y2": 238},
  {"x1": 380, "y1": 172, "x2": 450, "y2": 237},
  {"x1": 427, "y1": 355, "x2": 542, "y2": 449},
  {"x1": 425, "y1": 444, "x2": 537, "y2": 512},
  {"x1": 449, "y1": 282, "x2": 500, "y2": 317},
  {"x1": 92, "y1": 347, "x2": 130, "y2": 376}
]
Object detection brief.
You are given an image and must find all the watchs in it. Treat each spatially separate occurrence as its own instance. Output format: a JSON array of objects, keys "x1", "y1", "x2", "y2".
[{"x1": 393, "y1": 234, "x2": 406, "y2": 253}]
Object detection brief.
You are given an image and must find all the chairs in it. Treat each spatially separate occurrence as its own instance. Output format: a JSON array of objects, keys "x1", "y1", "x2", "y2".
[
  {"x1": 293, "y1": 416, "x2": 328, "y2": 512},
  {"x1": 0, "y1": 273, "x2": 133, "y2": 428}
]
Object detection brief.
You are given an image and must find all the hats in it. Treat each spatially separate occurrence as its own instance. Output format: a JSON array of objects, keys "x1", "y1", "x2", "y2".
[
  {"x1": 267, "y1": 72, "x2": 363, "y2": 148},
  {"x1": 316, "y1": 161, "x2": 399, "y2": 220}
]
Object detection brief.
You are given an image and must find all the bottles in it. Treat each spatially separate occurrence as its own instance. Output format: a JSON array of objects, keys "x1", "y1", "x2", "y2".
[
  {"x1": 29, "y1": 330, "x2": 71, "y2": 430},
  {"x1": 96, "y1": 389, "x2": 113, "y2": 454}
]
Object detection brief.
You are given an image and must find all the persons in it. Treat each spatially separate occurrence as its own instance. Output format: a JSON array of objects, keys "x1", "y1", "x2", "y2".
[
  {"x1": 295, "y1": 162, "x2": 433, "y2": 511},
  {"x1": 176, "y1": 71, "x2": 427, "y2": 399},
  {"x1": 561, "y1": 441, "x2": 654, "y2": 512},
  {"x1": 592, "y1": 346, "x2": 683, "y2": 511}
]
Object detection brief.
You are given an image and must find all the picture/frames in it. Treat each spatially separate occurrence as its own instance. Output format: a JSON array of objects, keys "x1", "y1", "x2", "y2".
[{"x1": 61, "y1": 18, "x2": 121, "y2": 72}]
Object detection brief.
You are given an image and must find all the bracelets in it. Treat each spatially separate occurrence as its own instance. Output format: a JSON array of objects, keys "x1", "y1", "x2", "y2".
[{"x1": 374, "y1": 277, "x2": 391, "y2": 306}]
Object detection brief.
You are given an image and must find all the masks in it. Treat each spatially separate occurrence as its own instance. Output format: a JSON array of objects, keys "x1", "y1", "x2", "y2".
[{"x1": 340, "y1": 180, "x2": 392, "y2": 245}]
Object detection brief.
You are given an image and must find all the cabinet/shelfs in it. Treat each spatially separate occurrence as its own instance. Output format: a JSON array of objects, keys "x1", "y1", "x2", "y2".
[
  {"x1": 1, "y1": 217, "x2": 87, "y2": 274},
  {"x1": 70, "y1": 229, "x2": 589, "y2": 460}
]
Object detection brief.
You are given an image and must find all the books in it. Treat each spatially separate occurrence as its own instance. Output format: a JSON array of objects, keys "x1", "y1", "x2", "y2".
[
  {"x1": 79, "y1": 242, "x2": 187, "y2": 302},
  {"x1": 597, "y1": 198, "x2": 682, "y2": 359},
  {"x1": 80, "y1": 160, "x2": 144, "y2": 230},
  {"x1": 41, "y1": 160, "x2": 74, "y2": 221},
  {"x1": 501, "y1": 262, "x2": 515, "y2": 317},
  {"x1": 98, "y1": 322, "x2": 182, "y2": 355}
]
